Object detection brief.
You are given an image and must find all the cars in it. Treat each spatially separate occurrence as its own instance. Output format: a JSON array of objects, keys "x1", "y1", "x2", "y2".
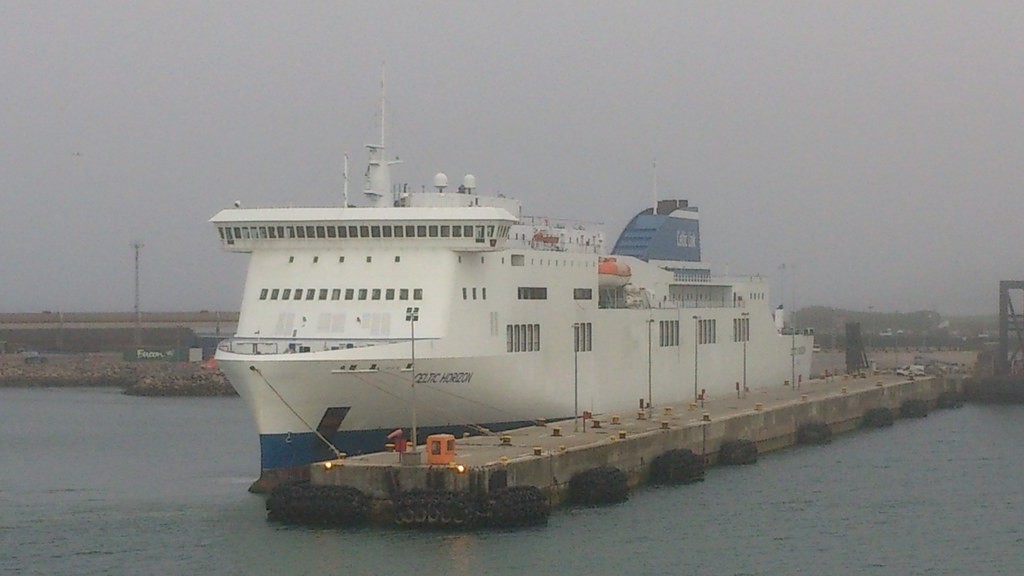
[{"x1": 896, "y1": 361, "x2": 959, "y2": 377}]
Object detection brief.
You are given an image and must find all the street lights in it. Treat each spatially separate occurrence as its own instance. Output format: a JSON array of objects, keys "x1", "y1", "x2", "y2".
[
  {"x1": 649, "y1": 318, "x2": 654, "y2": 418},
  {"x1": 692, "y1": 315, "x2": 697, "y2": 404},
  {"x1": 406, "y1": 306, "x2": 419, "y2": 450},
  {"x1": 896, "y1": 330, "x2": 903, "y2": 363}
]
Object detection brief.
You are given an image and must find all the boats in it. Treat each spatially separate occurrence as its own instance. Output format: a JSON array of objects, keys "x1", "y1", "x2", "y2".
[{"x1": 206, "y1": 59, "x2": 814, "y2": 493}]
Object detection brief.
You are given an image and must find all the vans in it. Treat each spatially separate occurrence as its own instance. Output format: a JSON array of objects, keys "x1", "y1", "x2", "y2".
[{"x1": 813, "y1": 343, "x2": 821, "y2": 353}]
